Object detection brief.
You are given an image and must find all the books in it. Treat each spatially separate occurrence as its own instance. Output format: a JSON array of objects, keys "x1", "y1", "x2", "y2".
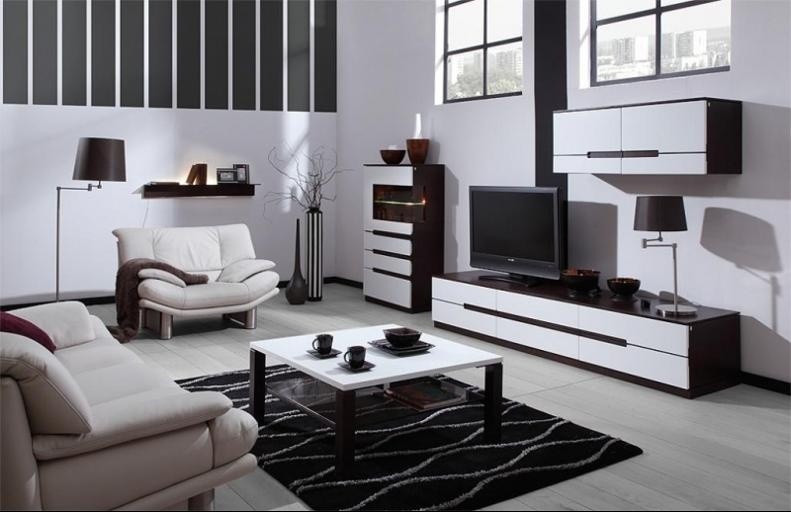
[{"x1": 149, "y1": 161, "x2": 208, "y2": 185}]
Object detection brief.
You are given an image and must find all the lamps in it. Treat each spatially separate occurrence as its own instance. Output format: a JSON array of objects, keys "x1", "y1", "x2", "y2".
[
  {"x1": 53, "y1": 135, "x2": 129, "y2": 303},
  {"x1": 633, "y1": 194, "x2": 700, "y2": 319}
]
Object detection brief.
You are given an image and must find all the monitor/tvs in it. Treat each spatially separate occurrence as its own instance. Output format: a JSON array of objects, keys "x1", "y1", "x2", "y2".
[{"x1": 469, "y1": 186, "x2": 561, "y2": 287}]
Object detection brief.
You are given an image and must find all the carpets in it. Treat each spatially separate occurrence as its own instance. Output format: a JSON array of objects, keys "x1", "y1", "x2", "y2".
[{"x1": 174, "y1": 361, "x2": 646, "y2": 512}]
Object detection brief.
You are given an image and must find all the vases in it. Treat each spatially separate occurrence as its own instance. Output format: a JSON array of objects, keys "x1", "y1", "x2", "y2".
[
  {"x1": 284, "y1": 217, "x2": 310, "y2": 305},
  {"x1": 379, "y1": 147, "x2": 407, "y2": 164}
]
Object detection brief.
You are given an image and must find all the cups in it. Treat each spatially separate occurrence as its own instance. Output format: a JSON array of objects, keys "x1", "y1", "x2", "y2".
[
  {"x1": 641, "y1": 298, "x2": 651, "y2": 310},
  {"x1": 312, "y1": 333, "x2": 333, "y2": 354},
  {"x1": 344, "y1": 345, "x2": 368, "y2": 368}
]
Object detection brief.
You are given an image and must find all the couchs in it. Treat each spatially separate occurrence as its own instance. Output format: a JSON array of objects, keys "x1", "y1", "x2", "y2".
[
  {"x1": 0, "y1": 297, "x2": 263, "y2": 511},
  {"x1": 107, "y1": 223, "x2": 281, "y2": 340}
]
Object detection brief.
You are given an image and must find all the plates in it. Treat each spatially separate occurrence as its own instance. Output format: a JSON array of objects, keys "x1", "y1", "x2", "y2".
[
  {"x1": 338, "y1": 360, "x2": 375, "y2": 374},
  {"x1": 370, "y1": 338, "x2": 435, "y2": 357},
  {"x1": 307, "y1": 348, "x2": 342, "y2": 360}
]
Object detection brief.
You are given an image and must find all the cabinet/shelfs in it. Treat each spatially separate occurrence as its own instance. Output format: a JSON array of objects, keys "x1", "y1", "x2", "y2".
[
  {"x1": 430, "y1": 269, "x2": 746, "y2": 401},
  {"x1": 551, "y1": 96, "x2": 743, "y2": 178},
  {"x1": 363, "y1": 164, "x2": 447, "y2": 314}
]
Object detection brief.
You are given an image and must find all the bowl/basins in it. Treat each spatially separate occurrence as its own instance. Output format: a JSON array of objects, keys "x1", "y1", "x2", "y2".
[
  {"x1": 608, "y1": 277, "x2": 640, "y2": 297},
  {"x1": 384, "y1": 326, "x2": 425, "y2": 346},
  {"x1": 562, "y1": 269, "x2": 601, "y2": 293},
  {"x1": 380, "y1": 147, "x2": 405, "y2": 165}
]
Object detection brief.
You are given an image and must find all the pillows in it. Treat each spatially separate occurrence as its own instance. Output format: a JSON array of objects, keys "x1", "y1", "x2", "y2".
[
  {"x1": 0, "y1": 311, "x2": 59, "y2": 356},
  {"x1": 0, "y1": 331, "x2": 95, "y2": 436}
]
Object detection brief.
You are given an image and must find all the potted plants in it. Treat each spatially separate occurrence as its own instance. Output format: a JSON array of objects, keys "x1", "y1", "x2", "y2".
[{"x1": 261, "y1": 129, "x2": 357, "y2": 302}]
